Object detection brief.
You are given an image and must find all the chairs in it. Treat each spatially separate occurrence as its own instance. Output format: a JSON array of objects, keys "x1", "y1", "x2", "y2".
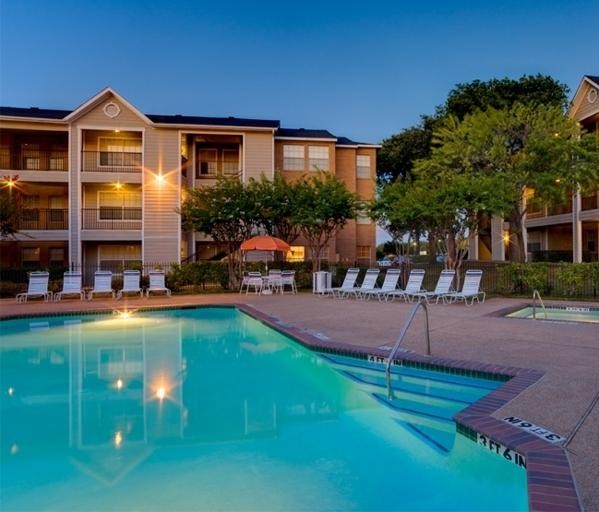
[
  {"x1": 87, "y1": 271, "x2": 115, "y2": 302},
  {"x1": 146, "y1": 271, "x2": 171, "y2": 298},
  {"x1": 53, "y1": 271, "x2": 85, "y2": 303},
  {"x1": 319, "y1": 268, "x2": 486, "y2": 307},
  {"x1": 117, "y1": 270, "x2": 143, "y2": 300},
  {"x1": 239, "y1": 269, "x2": 297, "y2": 296},
  {"x1": 16, "y1": 272, "x2": 53, "y2": 303}
]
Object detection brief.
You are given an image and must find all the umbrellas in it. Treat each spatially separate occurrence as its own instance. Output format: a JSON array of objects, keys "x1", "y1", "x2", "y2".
[{"x1": 240, "y1": 233, "x2": 292, "y2": 289}]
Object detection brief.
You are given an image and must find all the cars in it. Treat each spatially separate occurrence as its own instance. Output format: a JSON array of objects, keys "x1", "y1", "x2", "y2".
[
  {"x1": 436, "y1": 248, "x2": 468, "y2": 263},
  {"x1": 376, "y1": 254, "x2": 397, "y2": 267}
]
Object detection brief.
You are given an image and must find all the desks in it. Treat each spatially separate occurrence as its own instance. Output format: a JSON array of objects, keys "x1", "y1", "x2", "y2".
[{"x1": 312, "y1": 272, "x2": 332, "y2": 296}]
